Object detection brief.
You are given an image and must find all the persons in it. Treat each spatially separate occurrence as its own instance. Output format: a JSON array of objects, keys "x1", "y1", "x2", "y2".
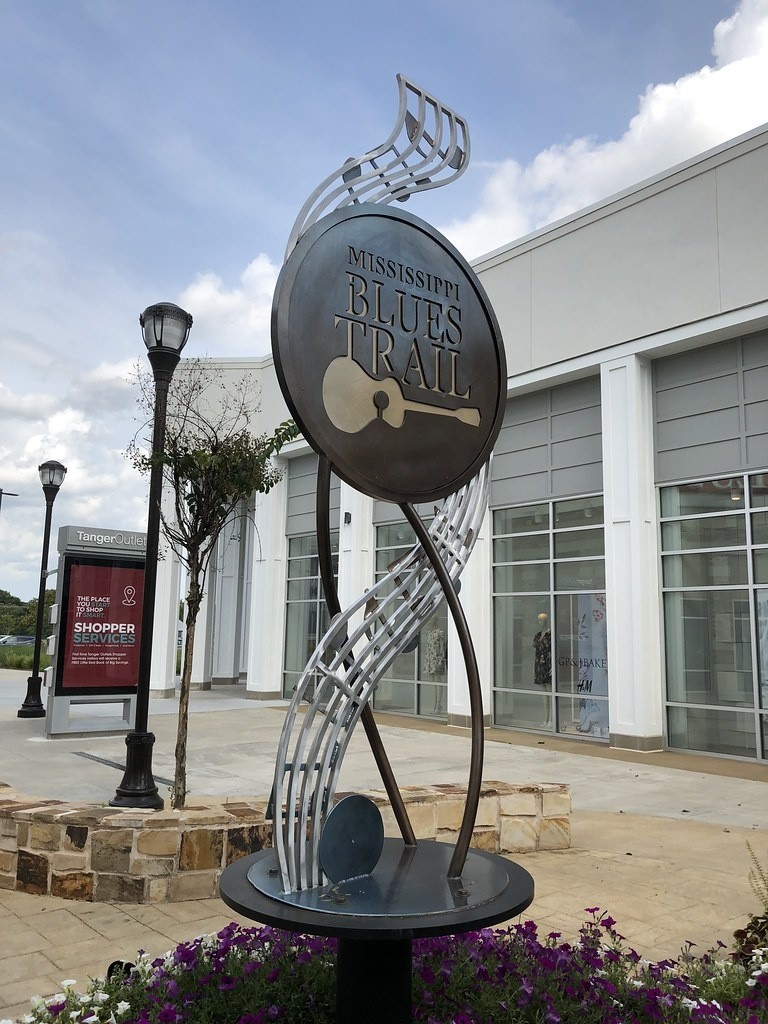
[
  {"x1": 531, "y1": 613, "x2": 551, "y2": 727},
  {"x1": 423, "y1": 613, "x2": 447, "y2": 715}
]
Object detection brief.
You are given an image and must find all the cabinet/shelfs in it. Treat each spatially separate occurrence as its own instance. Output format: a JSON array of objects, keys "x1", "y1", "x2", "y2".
[{"x1": 663, "y1": 487, "x2": 767, "y2": 748}]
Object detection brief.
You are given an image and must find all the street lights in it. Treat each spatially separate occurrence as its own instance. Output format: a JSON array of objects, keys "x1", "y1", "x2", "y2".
[
  {"x1": 15, "y1": 460, "x2": 68, "y2": 718},
  {"x1": 106, "y1": 300, "x2": 195, "y2": 811}
]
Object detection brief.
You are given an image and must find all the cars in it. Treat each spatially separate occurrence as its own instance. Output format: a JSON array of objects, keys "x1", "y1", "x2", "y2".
[{"x1": 0, "y1": 634, "x2": 46, "y2": 646}]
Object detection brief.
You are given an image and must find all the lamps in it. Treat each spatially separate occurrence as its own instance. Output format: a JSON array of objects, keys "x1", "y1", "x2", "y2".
[
  {"x1": 731, "y1": 482, "x2": 742, "y2": 499},
  {"x1": 535, "y1": 515, "x2": 544, "y2": 522},
  {"x1": 344, "y1": 512, "x2": 351, "y2": 524},
  {"x1": 585, "y1": 503, "x2": 592, "y2": 517}
]
